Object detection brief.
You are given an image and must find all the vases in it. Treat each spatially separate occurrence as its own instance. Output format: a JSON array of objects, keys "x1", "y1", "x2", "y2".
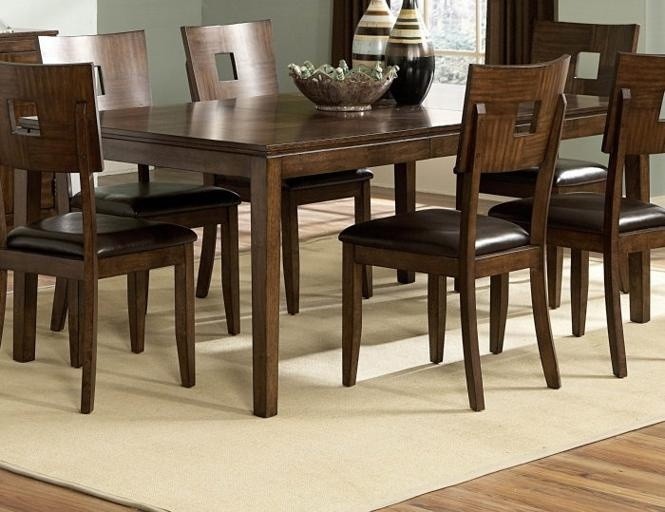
[{"x1": 351, "y1": 0, "x2": 397, "y2": 79}]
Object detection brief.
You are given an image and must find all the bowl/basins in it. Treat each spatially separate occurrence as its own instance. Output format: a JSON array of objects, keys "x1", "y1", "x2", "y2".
[{"x1": 288, "y1": 63, "x2": 399, "y2": 112}]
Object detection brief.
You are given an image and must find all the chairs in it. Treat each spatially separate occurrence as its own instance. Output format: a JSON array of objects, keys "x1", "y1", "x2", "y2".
[
  {"x1": 179, "y1": 17, "x2": 375, "y2": 321},
  {"x1": 445, "y1": 12, "x2": 645, "y2": 311},
  {"x1": 30, "y1": 28, "x2": 242, "y2": 358},
  {"x1": 332, "y1": 48, "x2": 574, "y2": 417},
  {"x1": 0, "y1": 57, "x2": 199, "y2": 418},
  {"x1": 480, "y1": 47, "x2": 665, "y2": 382}
]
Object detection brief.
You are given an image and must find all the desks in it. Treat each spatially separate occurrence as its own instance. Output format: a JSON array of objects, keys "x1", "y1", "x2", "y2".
[{"x1": 42, "y1": 81, "x2": 657, "y2": 422}]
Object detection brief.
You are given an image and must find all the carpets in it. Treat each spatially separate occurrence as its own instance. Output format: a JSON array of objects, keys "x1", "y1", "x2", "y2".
[{"x1": 2, "y1": 199, "x2": 665, "y2": 512}]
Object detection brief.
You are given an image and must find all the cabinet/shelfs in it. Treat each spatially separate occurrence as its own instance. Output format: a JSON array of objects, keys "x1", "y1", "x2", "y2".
[{"x1": 0, "y1": 26, "x2": 72, "y2": 234}]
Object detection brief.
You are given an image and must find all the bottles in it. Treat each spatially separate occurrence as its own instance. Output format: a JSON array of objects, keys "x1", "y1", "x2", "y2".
[
  {"x1": 288, "y1": 59, "x2": 400, "y2": 80},
  {"x1": 352, "y1": 0, "x2": 398, "y2": 109}
]
